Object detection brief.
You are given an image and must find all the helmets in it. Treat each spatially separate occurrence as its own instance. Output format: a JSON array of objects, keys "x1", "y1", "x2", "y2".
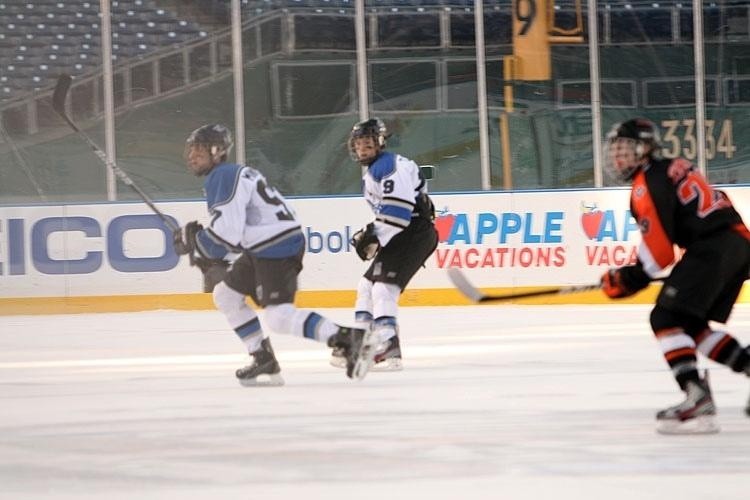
[
  {"x1": 348, "y1": 118, "x2": 387, "y2": 166},
  {"x1": 603, "y1": 118, "x2": 660, "y2": 183},
  {"x1": 185, "y1": 124, "x2": 234, "y2": 177}
]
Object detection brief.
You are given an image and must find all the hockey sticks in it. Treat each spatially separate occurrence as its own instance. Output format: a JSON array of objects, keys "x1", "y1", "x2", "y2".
[
  {"x1": 446, "y1": 264, "x2": 669, "y2": 303},
  {"x1": 355, "y1": 227, "x2": 376, "y2": 260},
  {"x1": 53, "y1": 75, "x2": 179, "y2": 232}
]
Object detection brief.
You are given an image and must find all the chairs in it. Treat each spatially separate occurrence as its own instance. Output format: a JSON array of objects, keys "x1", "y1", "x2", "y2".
[{"x1": 0, "y1": 0, "x2": 749, "y2": 116}]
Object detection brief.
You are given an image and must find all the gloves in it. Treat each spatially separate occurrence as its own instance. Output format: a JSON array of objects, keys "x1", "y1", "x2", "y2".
[
  {"x1": 350, "y1": 222, "x2": 380, "y2": 260},
  {"x1": 172, "y1": 220, "x2": 203, "y2": 255},
  {"x1": 600, "y1": 264, "x2": 652, "y2": 298}
]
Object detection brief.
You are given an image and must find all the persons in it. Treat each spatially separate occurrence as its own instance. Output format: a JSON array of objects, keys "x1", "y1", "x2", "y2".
[
  {"x1": 603, "y1": 119, "x2": 750, "y2": 429},
  {"x1": 172, "y1": 123, "x2": 366, "y2": 381},
  {"x1": 328, "y1": 118, "x2": 438, "y2": 372}
]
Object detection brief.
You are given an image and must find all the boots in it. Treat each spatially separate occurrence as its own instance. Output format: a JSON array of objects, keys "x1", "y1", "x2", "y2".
[
  {"x1": 328, "y1": 311, "x2": 402, "y2": 380},
  {"x1": 656, "y1": 368, "x2": 717, "y2": 421},
  {"x1": 235, "y1": 336, "x2": 281, "y2": 380}
]
